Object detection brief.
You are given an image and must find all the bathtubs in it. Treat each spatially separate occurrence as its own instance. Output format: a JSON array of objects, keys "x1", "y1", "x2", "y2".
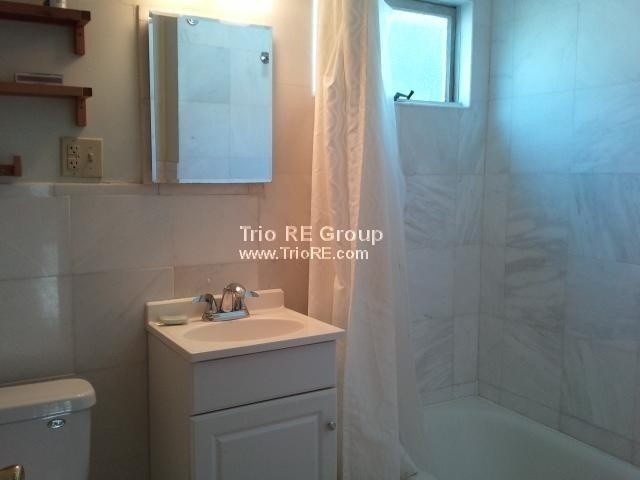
[{"x1": 389, "y1": 394, "x2": 640, "y2": 480}]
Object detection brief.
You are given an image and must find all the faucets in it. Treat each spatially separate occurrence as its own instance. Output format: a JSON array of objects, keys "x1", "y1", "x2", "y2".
[{"x1": 219, "y1": 282, "x2": 260, "y2": 313}]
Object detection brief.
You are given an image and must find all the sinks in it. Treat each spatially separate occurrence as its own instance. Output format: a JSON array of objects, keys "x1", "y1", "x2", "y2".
[{"x1": 183, "y1": 315, "x2": 305, "y2": 346}]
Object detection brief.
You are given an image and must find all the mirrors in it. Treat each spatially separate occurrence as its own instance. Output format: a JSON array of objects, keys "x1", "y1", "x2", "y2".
[{"x1": 148, "y1": 9, "x2": 273, "y2": 183}]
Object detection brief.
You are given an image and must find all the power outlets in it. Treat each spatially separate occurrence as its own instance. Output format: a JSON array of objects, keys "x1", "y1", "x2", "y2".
[{"x1": 61, "y1": 137, "x2": 103, "y2": 179}]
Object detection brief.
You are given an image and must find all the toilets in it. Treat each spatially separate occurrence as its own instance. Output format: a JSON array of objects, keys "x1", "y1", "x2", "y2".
[{"x1": 0, "y1": 373, "x2": 96, "y2": 480}]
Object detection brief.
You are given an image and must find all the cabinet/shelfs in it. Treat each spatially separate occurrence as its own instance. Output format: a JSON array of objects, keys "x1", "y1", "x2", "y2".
[
  {"x1": 0, "y1": 1, "x2": 92, "y2": 127},
  {"x1": 148, "y1": 344, "x2": 340, "y2": 479}
]
